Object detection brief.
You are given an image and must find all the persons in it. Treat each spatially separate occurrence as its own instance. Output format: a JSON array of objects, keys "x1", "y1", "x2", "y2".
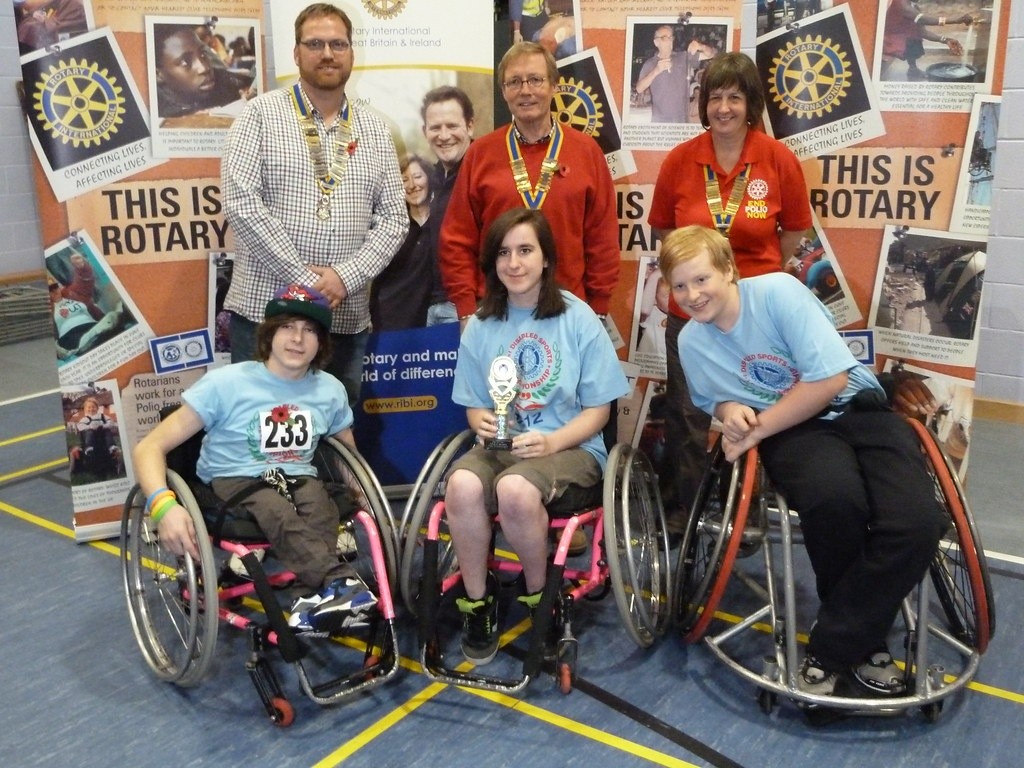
[
  {"x1": 12, "y1": 0, "x2": 88, "y2": 56},
  {"x1": 630, "y1": 24, "x2": 727, "y2": 123},
  {"x1": 370, "y1": 85, "x2": 476, "y2": 332},
  {"x1": 647, "y1": 50, "x2": 949, "y2": 712},
  {"x1": 445, "y1": 206, "x2": 630, "y2": 665},
  {"x1": 883, "y1": 0, "x2": 974, "y2": 76},
  {"x1": 875, "y1": 358, "x2": 937, "y2": 421},
  {"x1": 438, "y1": 42, "x2": 620, "y2": 555},
  {"x1": 130, "y1": 282, "x2": 378, "y2": 631},
  {"x1": 47, "y1": 253, "x2": 126, "y2": 358},
  {"x1": 509, "y1": 0, "x2": 578, "y2": 61},
  {"x1": 223, "y1": 4, "x2": 411, "y2": 580},
  {"x1": 636, "y1": 263, "x2": 671, "y2": 354},
  {"x1": 76, "y1": 398, "x2": 121, "y2": 461},
  {"x1": 153, "y1": 23, "x2": 257, "y2": 119}
]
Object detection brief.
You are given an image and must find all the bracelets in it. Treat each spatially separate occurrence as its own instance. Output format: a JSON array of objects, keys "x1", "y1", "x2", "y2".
[
  {"x1": 146, "y1": 487, "x2": 179, "y2": 522},
  {"x1": 938, "y1": 17, "x2": 946, "y2": 26},
  {"x1": 939, "y1": 35, "x2": 946, "y2": 43}
]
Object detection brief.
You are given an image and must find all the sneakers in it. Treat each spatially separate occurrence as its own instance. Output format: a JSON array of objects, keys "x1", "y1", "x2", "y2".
[
  {"x1": 787, "y1": 649, "x2": 845, "y2": 711},
  {"x1": 308, "y1": 578, "x2": 378, "y2": 632},
  {"x1": 850, "y1": 639, "x2": 908, "y2": 698},
  {"x1": 455, "y1": 568, "x2": 500, "y2": 666},
  {"x1": 555, "y1": 527, "x2": 587, "y2": 554},
  {"x1": 287, "y1": 588, "x2": 325, "y2": 632},
  {"x1": 516, "y1": 561, "x2": 571, "y2": 661}
]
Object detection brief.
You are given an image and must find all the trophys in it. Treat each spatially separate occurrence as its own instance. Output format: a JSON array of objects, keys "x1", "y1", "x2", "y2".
[{"x1": 484, "y1": 356, "x2": 518, "y2": 451}]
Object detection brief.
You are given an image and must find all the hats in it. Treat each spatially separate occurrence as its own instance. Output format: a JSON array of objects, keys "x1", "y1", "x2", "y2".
[{"x1": 265, "y1": 286, "x2": 332, "y2": 331}]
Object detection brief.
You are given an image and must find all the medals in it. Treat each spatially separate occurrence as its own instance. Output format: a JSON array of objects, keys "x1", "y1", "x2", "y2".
[{"x1": 317, "y1": 194, "x2": 332, "y2": 220}]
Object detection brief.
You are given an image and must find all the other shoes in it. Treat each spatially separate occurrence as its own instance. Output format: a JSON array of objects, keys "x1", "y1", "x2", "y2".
[
  {"x1": 707, "y1": 540, "x2": 759, "y2": 558},
  {"x1": 336, "y1": 531, "x2": 357, "y2": 557},
  {"x1": 230, "y1": 548, "x2": 265, "y2": 579},
  {"x1": 656, "y1": 528, "x2": 683, "y2": 551}
]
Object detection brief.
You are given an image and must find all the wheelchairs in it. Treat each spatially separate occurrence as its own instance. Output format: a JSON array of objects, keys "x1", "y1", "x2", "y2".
[
  {"x1": 117, "y1": 402, "x2": 402, "y2": 727},
  {"x1": 669, "y1": 415, "x2": 998, "y2": 726},
  {"x1": 401, "y1": 370, "x2": 672, "y2": 696}
]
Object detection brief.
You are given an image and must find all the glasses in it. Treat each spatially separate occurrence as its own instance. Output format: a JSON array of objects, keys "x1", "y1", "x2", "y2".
[
  {"x1": 655, "y1": 35, "x2": 674, "y2": 42},
  {"x1": 300, "y1": 39, "x2": 352, "y2": 53},
  {"x1": 49, "y1": 283, "x2": 59, "y2": 292},
  {"x1": 503, "y1": 75, "x2": 549, "y2": 90}
]
